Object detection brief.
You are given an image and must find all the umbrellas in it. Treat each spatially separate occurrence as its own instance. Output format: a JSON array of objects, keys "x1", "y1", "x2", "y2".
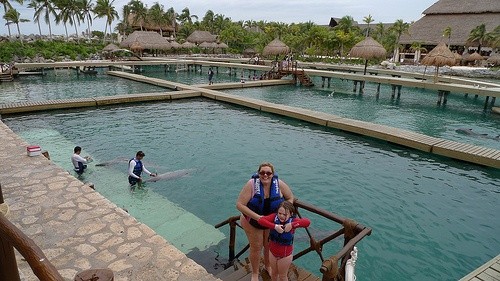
[
  {"x1": 262, "y1": 38, "x2": 290, "y2": 61},
  {"x1": 171, "y1": 41, "x2": 229, "y2": 56},
  {"x1": 422, "y1": 42, "x2": 500, "y2": 82},
  {"x1": 103, "y1": 41, "x2": 143, "y2": 58},
  {"x1": 244, "y1": 47, "x2": 259, "y2": 58},
  {"x1": 348, "y1": 36, "x2": 386, "y2": 76}
]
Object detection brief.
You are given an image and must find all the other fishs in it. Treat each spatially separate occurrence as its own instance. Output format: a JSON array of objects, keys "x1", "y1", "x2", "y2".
[
  {"x1": 96, "y1": 156, "x2": 162, "y2": 168},
  {"x1": 455, "y1": 128, "x2": 500, "y2": 142},
  {"x1": 143, "y1": 167, "x2": 207, "y2": 183},
  {"x1": 329, "y1": 90, "x2": 335, "y2": 97}
]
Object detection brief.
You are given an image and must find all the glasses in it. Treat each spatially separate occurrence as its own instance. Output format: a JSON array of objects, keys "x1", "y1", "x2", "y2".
[{"x1": 258, "y1": 171, "x2": 272, "y2": 176}]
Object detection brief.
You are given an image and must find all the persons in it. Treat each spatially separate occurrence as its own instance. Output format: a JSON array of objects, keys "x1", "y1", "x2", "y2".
[
  {"x1": 241, "y1": 66, "x2": 279, "y2": 83},
  {"x1": 283, "y1": 54, "x2": 294, "y2": 68},
  {"x1": 254, "y1": 55, "x2": 258, "y2": 65},
  {"x1": 89, "y1": 52, "x2": 102, "y2": 61},
  {"x1": 236, "y1": 162, "x2": 294, "y2": 281},
  {"x1": 72, "y1": 146, "x2": 88, "y2": 175},
  {"x1": 208, "y1": 67, "x2": 213, "y2": 85},
  {"x1": 127, "y1": 151, "x2": 157, "y2": 188},
  {"x1": 259, "y1": 200, "x2": 311, "y2": 281},
  {"x1": 36, "y1": 51, "x2": 46, "y2": 62},
  {"x1": 1, "y1": 54, "x2": 31, "y2": 73}
]
